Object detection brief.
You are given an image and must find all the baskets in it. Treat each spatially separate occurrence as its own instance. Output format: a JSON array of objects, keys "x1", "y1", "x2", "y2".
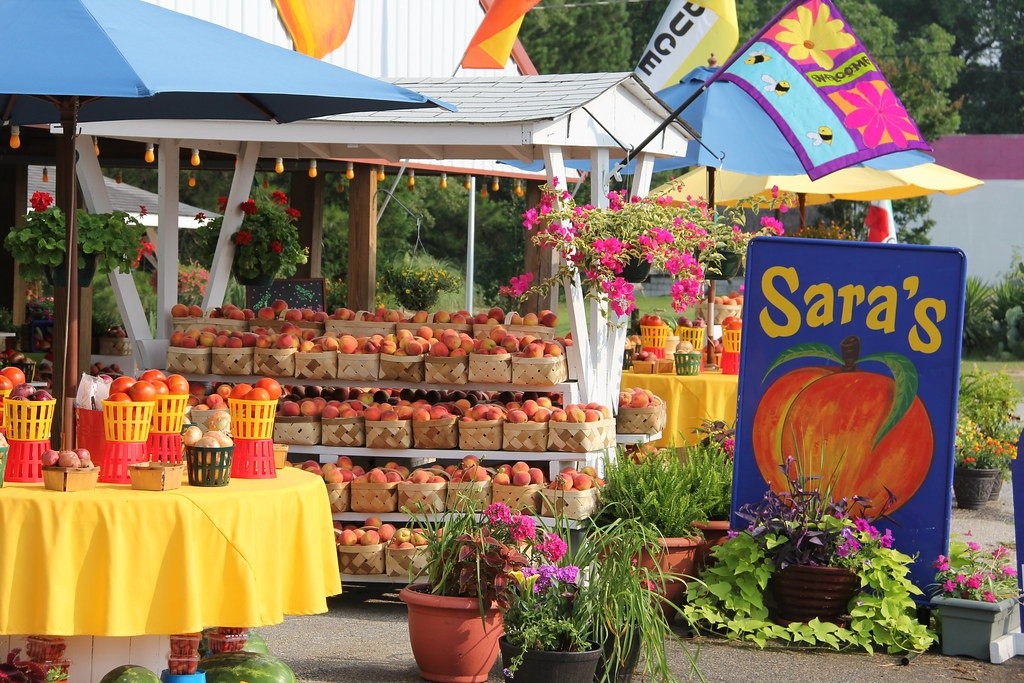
[{"x1": 0, "y1": 318, "x2": 741, "y2": 576}]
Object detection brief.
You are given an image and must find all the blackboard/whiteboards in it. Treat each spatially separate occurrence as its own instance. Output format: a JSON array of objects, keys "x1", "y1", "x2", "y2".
[{"x1": 247, "y1": 278, "x2": 326, "y2": 312}]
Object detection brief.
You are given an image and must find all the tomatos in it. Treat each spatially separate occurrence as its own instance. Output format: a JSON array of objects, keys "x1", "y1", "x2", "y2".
[
  {"x1": 639, "y1": 314, "x2": 666, "y2": 347},
  {"x1": 721, "y1": 316, "x2": 743, "y2": 346},
  {"x1": 228, "y1": 377, "x2": 283, "y2": 437},
  {"x1": 105, "y1": 369, "x2": 189, "y2": 439}
]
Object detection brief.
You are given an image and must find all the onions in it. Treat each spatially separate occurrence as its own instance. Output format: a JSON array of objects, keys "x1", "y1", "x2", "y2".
[
  {"x1": 184, "y1": 411, "x2": 233, "y2": 483},
  {"x1": 7, "y1": 353, "x2": 34, "y2": 371},
  {"x1": 677, "y1": 316, "x2": 705, "y2": 340},
  {"x1": 42, "y1": 448, "x2": 95, "y2": 488},
  {"x1": 676, "y1": 341, "x2": 701, "y2": 373},
  {"x1": 8, "y1": 383, "x2": 54, "y2": 438}
]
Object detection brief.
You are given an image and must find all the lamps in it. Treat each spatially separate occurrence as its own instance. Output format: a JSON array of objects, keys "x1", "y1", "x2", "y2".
[{"x1": 482, "y1": 178, "x2": 488, "y2": 198}]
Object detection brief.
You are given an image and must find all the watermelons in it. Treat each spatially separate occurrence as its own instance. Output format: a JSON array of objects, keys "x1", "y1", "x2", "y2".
[
  {"x1": 99, "y1": 665, "x2": 164, "y2": 683},
  {"x1": 197, "y1": 628, "x2": 297, "y2": 683}
]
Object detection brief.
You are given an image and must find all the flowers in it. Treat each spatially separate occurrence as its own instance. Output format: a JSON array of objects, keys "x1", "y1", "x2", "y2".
[
  {"x1": 26, "y1": 295, "x2": 54, "y2": 320},
  {"x1": 144, "y1": 263, "x2": 209, "y2": 296},
  {"x1": 10, "y1": 190, "x2": 156, "y2": 282},
  {"x1": 937, "y1": 540, "x2": 1018, "y2": 604},
  {"x1": 690, "y1": 249, "x2": 742, "y2": 281},
  {"x1": 517, "y1": 558, "x2": 596, "y2": 647},
  {"x1": 178, "y1": 189, "x2": 310, "y2": 279},
  {"x1": 685, "y1": 465, "x2": 931, "y2": 661},
  {"x1": 382, "y1": 265, "x2": 463, "y2": 291},
  {"x1": 956, "y1": 371, "x2": 1020, "y2": 464},
  {"x1": 504, "y1": 177, "x2": 797, "y2": 317},
  {"x1": 391, "y1": 490, "x2": 568, "y2": 594}
]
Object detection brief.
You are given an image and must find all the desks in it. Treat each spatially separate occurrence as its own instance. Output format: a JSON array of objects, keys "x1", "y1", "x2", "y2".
[
  {"x1": 621, "y1": 366, "x2": 739, "y2": 453},
  {"x1": 1, "y1": 467, "x2": 343, "y2": 683}
]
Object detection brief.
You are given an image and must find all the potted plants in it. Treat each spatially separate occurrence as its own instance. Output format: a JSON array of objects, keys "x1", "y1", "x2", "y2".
[
  {"x1": 604, "y1": 448, "x2": 701, "y2": 617},
  {"x1": 687, "y1": 442, "x2": 733, "y2": 574},
  {"x1": 579, "y1": 516, "x2": 668, "y2": 683}
]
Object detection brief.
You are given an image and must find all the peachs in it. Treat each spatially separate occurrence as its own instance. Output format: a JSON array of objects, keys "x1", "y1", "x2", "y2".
[
  {"x1": 1, "y1": 334, "x2": 53, "y2": 360},
  {"x1": 276, "y1": 385, "x2": 659, "y2": 549},
  {"x1": 107, "y1": 325, "x2": 127, "y2": 338},
  {"x1": 711, "y1": 290, "x2": 746, "y2": 307},
  {"x1": 165, "y1": 298, "x2": 571, "y2": 359},
  {"x1": 185, "y1": 384, "x2": 233, "y2": 411},
  {"x1": 625, "y1": 334, "x2": 658, "y2": 362}
]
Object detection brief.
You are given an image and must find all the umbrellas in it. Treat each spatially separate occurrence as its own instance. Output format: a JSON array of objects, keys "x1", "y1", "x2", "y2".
[
  {"x1": 645, "y1": 160, "x2": 984, "y2": 244},
  {"x1": 0, "y1": 0, "x2": 458, "y2": 453},
  {"x1": 495, "y1": 50, "x2": 936, "y2": 366}
]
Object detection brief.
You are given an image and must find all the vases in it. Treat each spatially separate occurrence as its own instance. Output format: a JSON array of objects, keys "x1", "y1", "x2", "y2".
[
  {"x1": 930, "y1": 593, "x2": 1022, "y2": 663},
  {"x1": 496, "y1": 633, "x2": 604, "y2": 683},
  {"x1": 953, "y1": 463, "x2": 1006, "y2": 508},
  {"x1": 396, "y1": 584, "x2": 508, "y2": 683},
  {"x1": 773, "y1": 560, "x2": 855, "y2": 625},
  {"x1": 394, "y1": 287, "x2": 437, "y2": 311},
  {"x1": 235, "y1": 257, "x2": 279, "y2": 288},
  {"x1": 46, "y1": 251, "x2": 100, "y2": 287},
  {"x1": 606, "y1": 243, "x2": 651, "y2": 282}
]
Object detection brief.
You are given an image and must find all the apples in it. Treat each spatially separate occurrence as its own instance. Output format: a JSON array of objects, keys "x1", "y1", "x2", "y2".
[{"x1": 91, "y1": 362, "x2": 123, "y2": 374}]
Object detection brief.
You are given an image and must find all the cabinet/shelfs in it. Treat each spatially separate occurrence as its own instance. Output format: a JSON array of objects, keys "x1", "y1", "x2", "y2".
[{"x1": 155, "y1": 318, "x2": 661, "y2": 585}]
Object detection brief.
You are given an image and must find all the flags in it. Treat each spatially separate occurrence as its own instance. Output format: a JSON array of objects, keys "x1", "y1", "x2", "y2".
[{"x1": 704, "y1": 0, "x2": 935, "y2": 184}]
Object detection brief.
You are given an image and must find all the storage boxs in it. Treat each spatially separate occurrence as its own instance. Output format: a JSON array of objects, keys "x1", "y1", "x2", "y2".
[
  {"x1": 129, "y1": 461, "x2": 185, "y2": 491},
  {"x1": 167, "y1": 657, "x2": 202, "y2": 674},
  {"x1": 98, "y1": 336, "x2": 133, "y2": 356},
  {"x1": 208, "y1": 633, "x2": 249, "y2": 651},
  {"x1": 32, "y1": 661, "x2": 70, "y2": 680},
  {"x1": 169, "y1": 633, "x2": 203, "y2": 655},
  {"x1": 40, "y1": 466, "x2": 101, "y2": 493},
  {"x1": 25, "y1": 634, "x2": 68, "y2": 659},
  {"x1": 21, "y1": 320, "x2": 53, "y2": 353},
  {"x1": 1, "y1": 360, "x2": 36, "y2": 383}
]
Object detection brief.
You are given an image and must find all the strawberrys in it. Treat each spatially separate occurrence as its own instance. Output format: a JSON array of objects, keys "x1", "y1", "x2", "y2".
[
  {"x1": 24, "y1": 636, "x2": 71, "y2": 681},
  {"x1": 166, "y1": 626, "x2": 249, "y2": 675}
]
